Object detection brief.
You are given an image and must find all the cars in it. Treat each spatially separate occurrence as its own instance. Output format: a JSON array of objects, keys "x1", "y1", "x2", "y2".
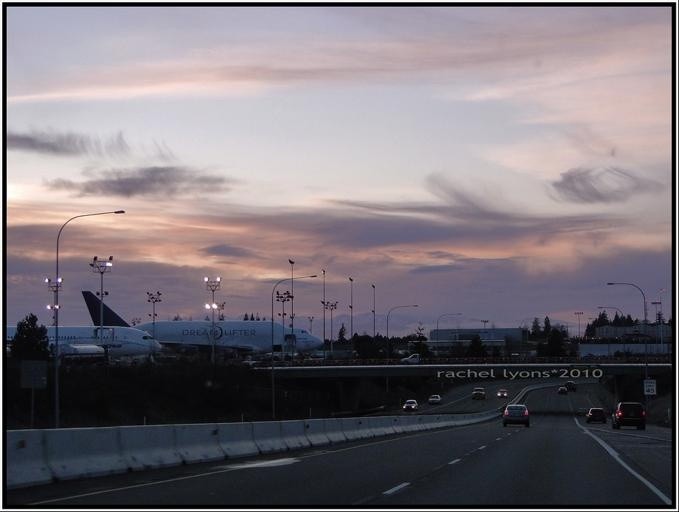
[
  {"x1": 472, "y1": 387, "x2": 485, "y2": 400},
  {"x1": 497, "y1": 389, "x2": 508, "y2": 399},
  {"x1": 557, "y1": 381, "x2": 576, "y2": 395},
  {"x1": 428, "y1": 394, "x2": 443, "y2": 405},
  {"x1": 585, "y1": 401, "x2": 647, "y2": 430},
  {"x1": 503, "y1": 404, "x2": 530, "y2": 427}
]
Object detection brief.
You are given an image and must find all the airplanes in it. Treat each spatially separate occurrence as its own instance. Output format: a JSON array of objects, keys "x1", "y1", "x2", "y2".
[{"x1": 7, "y1": 290, "x2": 325, "y2": 359}]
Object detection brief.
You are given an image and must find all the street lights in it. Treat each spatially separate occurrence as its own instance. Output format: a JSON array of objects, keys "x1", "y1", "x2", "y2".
[
  {"x1": 53, "y1": 207, "x2": 420, "y2": 428},
  {"x1": 572, "y1": 280, "x2": 649, "y2": 365},
  {"x1": 434, "y1": 311, "x2": 493, "y2": 362}
]
